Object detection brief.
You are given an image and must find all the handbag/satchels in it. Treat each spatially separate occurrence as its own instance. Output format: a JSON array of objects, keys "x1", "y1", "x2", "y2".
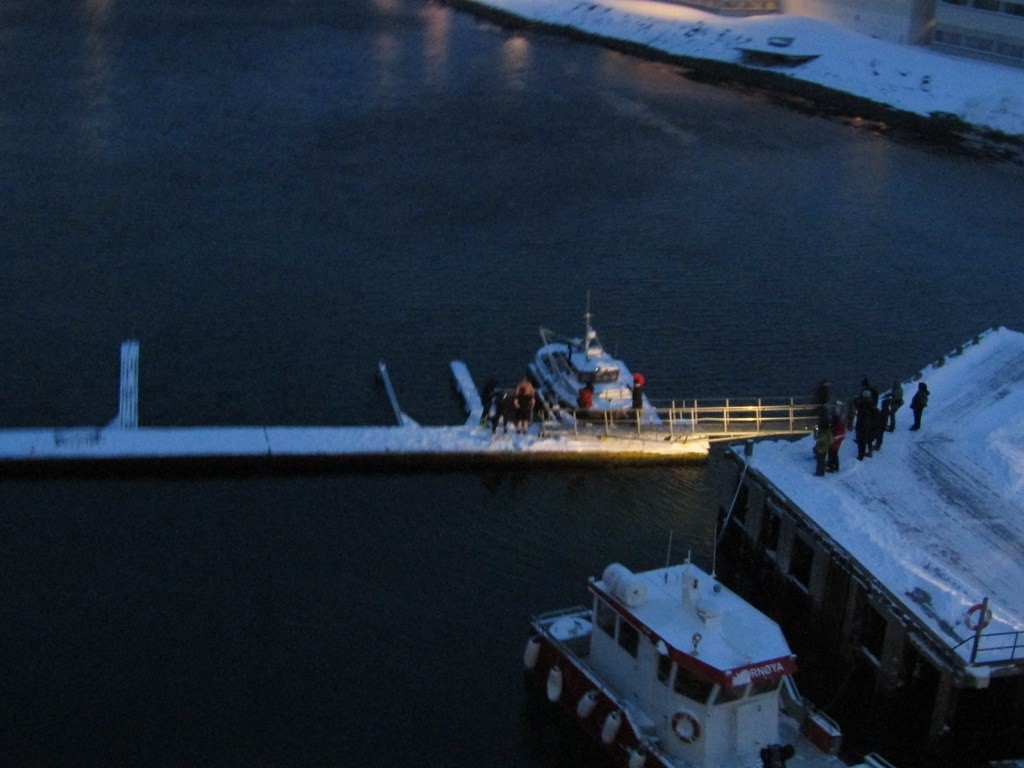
[
  {"x1": 534, "y1": 390, "x2": 546, "y2": 408},
  {"x1": 895, "y1": 399, "x2": 904, "y2": 405}
]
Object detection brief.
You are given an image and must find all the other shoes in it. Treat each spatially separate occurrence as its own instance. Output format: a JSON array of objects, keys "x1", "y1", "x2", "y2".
[{"x1": 813, "y1": 425, "x2": 922, "y2": 477}]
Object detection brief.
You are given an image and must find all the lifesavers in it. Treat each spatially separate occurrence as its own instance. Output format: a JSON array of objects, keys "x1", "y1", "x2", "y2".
[
  {"x1": 671, "y1": 710, "x2": 702, "y2": 745},
  {"x1": 963, "y1": 604, "x2": 993, "y2": 631}
]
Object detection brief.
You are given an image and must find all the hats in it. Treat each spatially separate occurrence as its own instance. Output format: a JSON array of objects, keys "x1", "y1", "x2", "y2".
[
  {"x1": 832, "y1": 415, "x2": 838, "y2": 423},
  {"x1": 862, "y1": 390, "x2": 871, "y2": 398},
  {"x1": 862, "y1": 378, "x2": 868, "y2": 386}
]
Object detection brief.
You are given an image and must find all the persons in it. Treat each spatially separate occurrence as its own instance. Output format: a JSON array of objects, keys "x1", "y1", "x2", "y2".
[
  {"x1": 577, "y1": 383, "x2": 594, "y2": 426},
  {"x1": 813, "y1": 378, "x2": 930, "y2": 477},
  {"x1": 626, "y1": 374, "x2": 644, "y2": 427},
  {"x1": 479, "y1": 372, "x2": 563, "y2": 435}
]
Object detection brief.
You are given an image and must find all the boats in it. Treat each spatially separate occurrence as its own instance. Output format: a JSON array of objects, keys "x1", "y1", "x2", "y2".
[
  {"x1": 535, "y1": 325, "x2": 635, "y2": 420},
  {"x1": 524, "y1": 560, "x2": 895, "y2": 767}
]
